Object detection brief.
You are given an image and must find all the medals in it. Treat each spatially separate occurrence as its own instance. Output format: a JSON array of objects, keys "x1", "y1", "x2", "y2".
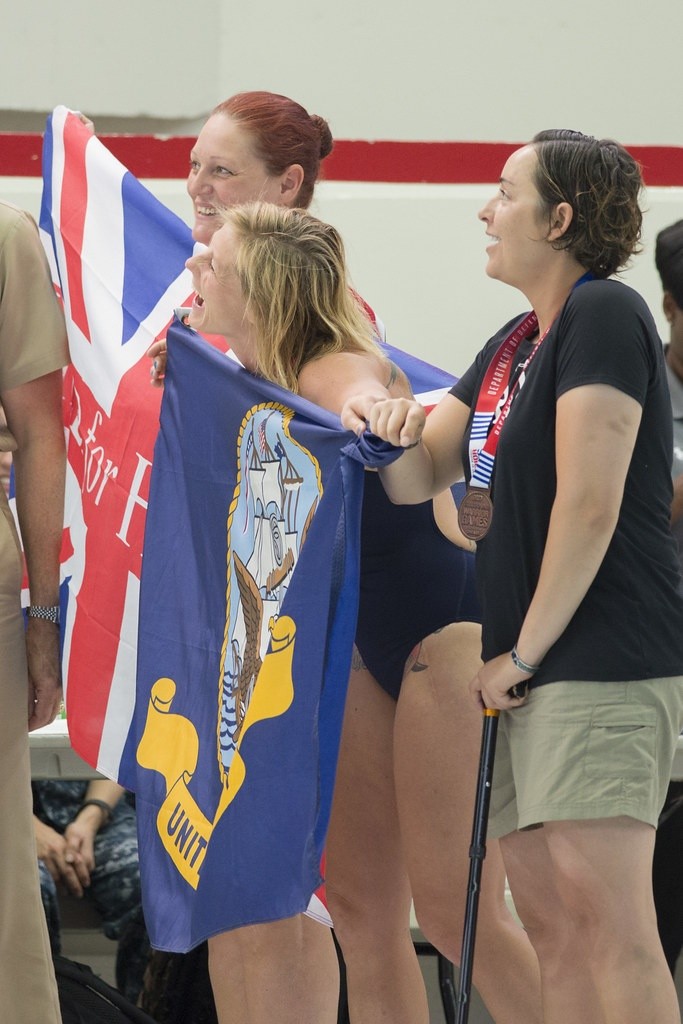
[{"x1": 455, "y1": 488, "x2": 492, "y2": 541}]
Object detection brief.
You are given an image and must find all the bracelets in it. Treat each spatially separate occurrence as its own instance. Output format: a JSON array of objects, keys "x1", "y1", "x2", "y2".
[
  {"x1": 75, "y1": 799, "x2": 112, "y2": 822},
  {"x1": 510, "y1": 647, "x2": 540, "y2": 680}
]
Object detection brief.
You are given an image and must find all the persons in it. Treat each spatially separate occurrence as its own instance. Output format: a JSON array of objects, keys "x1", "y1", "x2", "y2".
[
  {"x1": 374, "y1": 130, "x2": 682, "y2": 1024},
  {"x1": 651, "y1": 220, "x2": 683, "y2": 528},
  {"x1": 0, "y1": 203, "x2": 75, "y2": 1024},
  {"x1": 28, "y1": 776, "x2": 152, "y2": 1010},
  {"x1": 185, "y1": 203, "x2": 535, "y2": 1024},
  {"x1": 43, "y1": 87, "x2": 379, "y2": 1024}
]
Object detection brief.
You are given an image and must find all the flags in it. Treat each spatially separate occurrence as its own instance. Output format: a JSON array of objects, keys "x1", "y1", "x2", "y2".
[
  {"x1": 114, "y1": 321, "x2": 406, "y2": 955},
  {"x1": 24, "y1": 99, "x2": 461, "y2": 787}
]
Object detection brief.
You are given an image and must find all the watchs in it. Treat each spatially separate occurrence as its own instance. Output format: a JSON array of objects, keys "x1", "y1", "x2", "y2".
[{"x1": 28, "y1": 604, "x2": 64, "y2": 626}]
